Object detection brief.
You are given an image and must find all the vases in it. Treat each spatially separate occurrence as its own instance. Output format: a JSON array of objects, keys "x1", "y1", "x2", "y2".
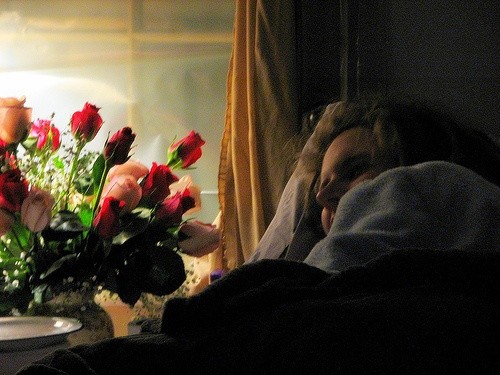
[
  {"x1": 23, "y1": 301, "x2": 115, "y2": 346},
  {"x1": 0, "y1": 316, "x2": 83, "y2": 375}
]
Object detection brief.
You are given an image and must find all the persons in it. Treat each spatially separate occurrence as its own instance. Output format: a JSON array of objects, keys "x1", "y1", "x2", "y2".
[{"x1": 17, "y1": 95, "x2": 500, "y2": 375}]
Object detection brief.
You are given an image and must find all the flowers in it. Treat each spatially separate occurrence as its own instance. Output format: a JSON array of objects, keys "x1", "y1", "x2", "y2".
[{"x1": 0, "y1": 95, "x2": 225, "y2": 309}]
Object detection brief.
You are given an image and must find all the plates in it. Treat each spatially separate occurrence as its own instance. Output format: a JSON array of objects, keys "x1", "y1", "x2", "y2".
[{"x1": 0, "y1": 316, "x2": 83, "y2": 352}]
